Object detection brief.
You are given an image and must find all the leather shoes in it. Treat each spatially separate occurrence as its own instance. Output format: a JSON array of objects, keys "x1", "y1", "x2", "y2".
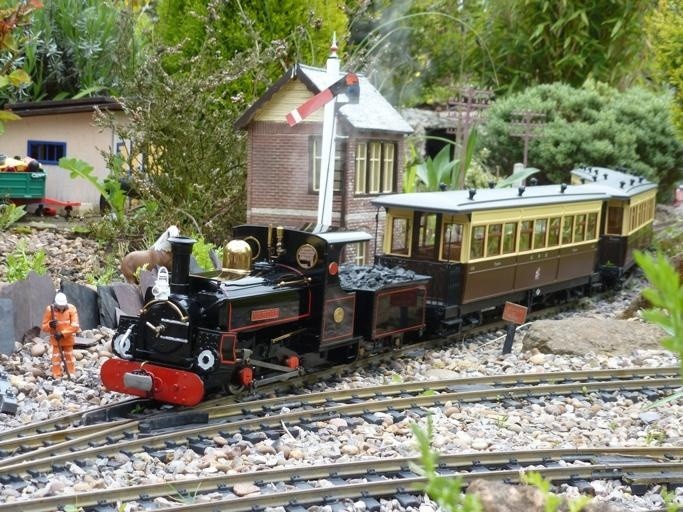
[
  {"x1": 48, "y1": 320, "x2": 56, "y2": 329},
  {"x1": 54, "y1": 331, "x2": 63, "y2": 341}
]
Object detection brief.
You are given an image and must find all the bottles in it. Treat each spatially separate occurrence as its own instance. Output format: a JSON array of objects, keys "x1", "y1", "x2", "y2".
[{"x1": 53, "y1": 292, "x2": 68, "y2": 306}]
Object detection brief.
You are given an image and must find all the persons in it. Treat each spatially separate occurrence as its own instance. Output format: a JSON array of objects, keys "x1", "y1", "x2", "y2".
[{"x1": 39, "y1": 292, "x2": 79, "y2": 382}]
